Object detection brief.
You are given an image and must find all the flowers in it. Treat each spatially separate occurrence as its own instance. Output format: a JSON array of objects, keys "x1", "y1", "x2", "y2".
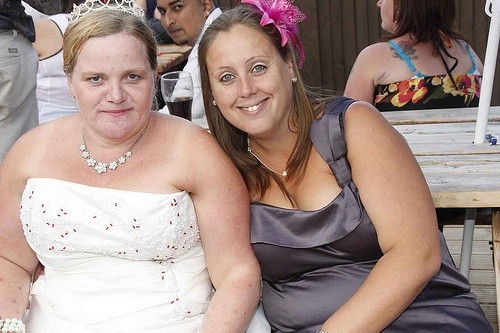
[{"x1": 241, "y1": 0, "x2": 313, "y2": 70}]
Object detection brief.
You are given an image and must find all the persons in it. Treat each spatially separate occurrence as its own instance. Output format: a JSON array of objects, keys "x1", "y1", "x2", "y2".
[
  {"x1": 0, "y1": 0, "x2": 264, "y2": 333},
  {"x1": 135, "y1": 0, "x2": 223, "y2": 135},
  {"x1": 197, "y1": 0, "x2": 494, "y2": 333},
  {"x1": 19, "y1": 0, "x2": 157, "y2": 124},
  {"x1": 0, "y1": 0, "x2": 39, "y2": 162},
  {"x1": 343, "y1": 0, "x2": 485, "y2": 230}
]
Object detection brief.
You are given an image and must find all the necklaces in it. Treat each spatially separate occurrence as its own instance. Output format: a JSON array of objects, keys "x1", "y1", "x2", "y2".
[
  {"x1": 80, "y1": 114, "x2": 150, "y2": 174},
  {"x1": 246, "y1": 129, "x2": 304, "y2": 177}
]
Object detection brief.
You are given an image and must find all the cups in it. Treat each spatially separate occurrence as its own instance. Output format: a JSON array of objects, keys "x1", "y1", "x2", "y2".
[{"x1": 161, "y1": 71, "x2": 193, "y2": 122}]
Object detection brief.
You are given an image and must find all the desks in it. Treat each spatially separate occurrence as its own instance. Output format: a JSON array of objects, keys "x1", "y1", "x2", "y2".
[
  {"x1": 156, "y1": 44, "x2": 192, "y2": 73},
  {"x1": 380, "y1": 105, "x2": 500, "y2": 207}
]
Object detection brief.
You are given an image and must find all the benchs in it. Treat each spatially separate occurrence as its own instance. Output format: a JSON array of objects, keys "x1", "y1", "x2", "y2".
[{"x1": 442, "y1": 225, "x2": 500, "y2": 333}]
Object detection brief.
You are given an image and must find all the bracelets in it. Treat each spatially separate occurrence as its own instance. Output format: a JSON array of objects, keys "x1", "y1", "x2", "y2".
[{"x1": 0, "y1": 318, "x2": 25, "y2": 333}]
